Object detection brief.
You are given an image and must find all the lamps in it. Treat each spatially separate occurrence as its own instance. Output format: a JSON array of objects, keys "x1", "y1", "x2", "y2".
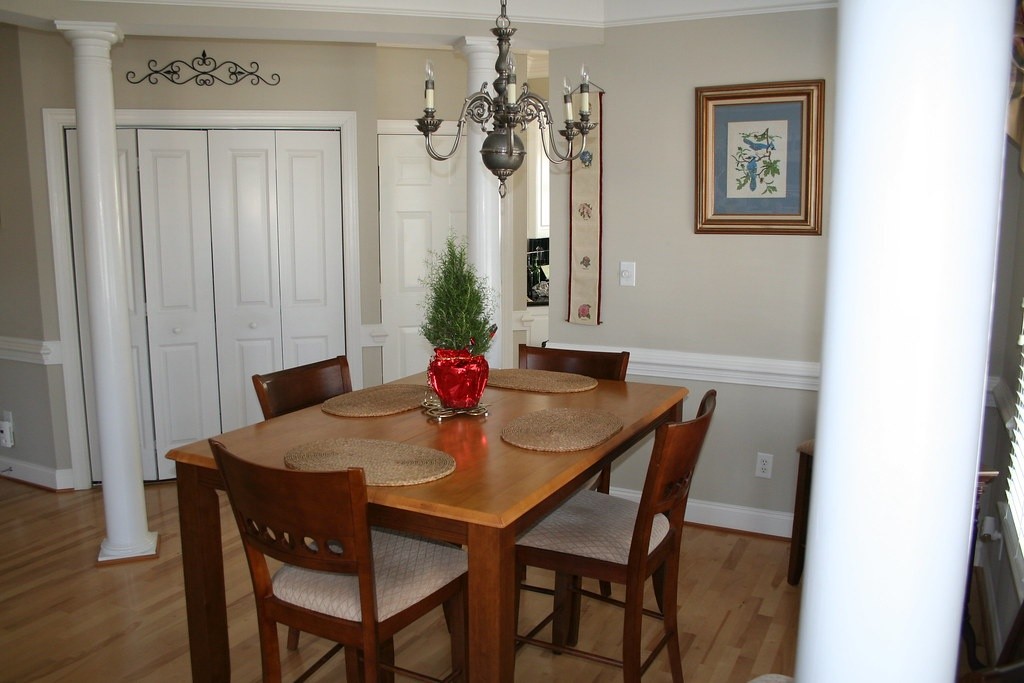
[{"x1": 414, "y1": 1, "x2": 597, "y2": 198}]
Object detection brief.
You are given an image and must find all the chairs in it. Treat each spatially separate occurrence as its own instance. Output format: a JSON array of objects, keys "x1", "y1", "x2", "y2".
[
  {"x1": 208, "y1": 437, "x2": 468, "y2": 683},
  {"x1": 252, "y1": 355, "x2": 353, "y2": 651},
  {"x1": 518, "y1": 343, "x2": 630, "y2": 647}
]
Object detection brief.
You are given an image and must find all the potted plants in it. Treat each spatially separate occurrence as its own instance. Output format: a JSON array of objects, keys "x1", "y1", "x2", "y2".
[{"x1": 416, "y1": 224, "x2": 498, "y2": 409}]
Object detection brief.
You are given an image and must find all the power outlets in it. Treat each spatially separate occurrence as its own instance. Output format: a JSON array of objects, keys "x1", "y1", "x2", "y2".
[
  {"x1": 754, "y1": 452, "x2": 773, "y2": 479},
  {"x1": 0, "y1": 422, "x2": 14, "y2": 448}
]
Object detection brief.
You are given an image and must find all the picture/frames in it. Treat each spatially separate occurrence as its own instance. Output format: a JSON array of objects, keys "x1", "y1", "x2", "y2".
[{"x1": 694, "y1": 79, "x2": 825, "y2": 236}]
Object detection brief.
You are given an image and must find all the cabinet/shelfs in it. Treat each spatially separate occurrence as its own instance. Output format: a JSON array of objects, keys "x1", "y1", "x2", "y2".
[{"x1": 65, "y1": 129, "x2": 346, "y2": 482}]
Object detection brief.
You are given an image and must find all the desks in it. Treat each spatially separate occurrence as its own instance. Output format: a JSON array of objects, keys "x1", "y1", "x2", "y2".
[
  {"x1": 165, "y1": 367, "x2": 689, "y2": 683},
  {"x1": 513, "y1": 389, "x2": 717, "y2": 682}
]
